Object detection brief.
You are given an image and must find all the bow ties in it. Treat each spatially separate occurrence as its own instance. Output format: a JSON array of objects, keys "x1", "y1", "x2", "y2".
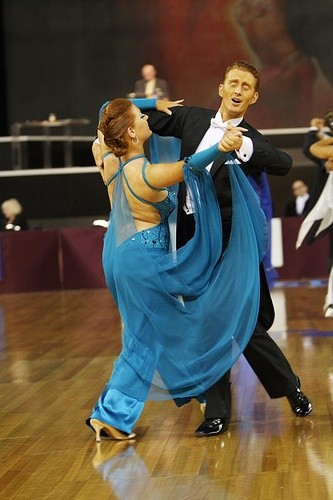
[{"x1": 210, "y1": 118, "x2": 232, "y2": 131}]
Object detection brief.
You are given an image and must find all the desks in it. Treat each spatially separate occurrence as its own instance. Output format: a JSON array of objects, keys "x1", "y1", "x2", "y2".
[{"x1": 21, "y1": 120, "x2": 88, "y2": 170}]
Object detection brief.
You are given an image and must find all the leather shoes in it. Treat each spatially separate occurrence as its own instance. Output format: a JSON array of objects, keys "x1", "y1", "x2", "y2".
[
  {"x1": 195, "y1": 417, "x2": 230, "y2": 437},
  {"x1": 286, "y1": 387, "x2": 312, "y2": 418}
]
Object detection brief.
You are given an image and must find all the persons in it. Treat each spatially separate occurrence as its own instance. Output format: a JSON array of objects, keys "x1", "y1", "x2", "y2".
[{"x1": 0, "y1": 60, "x2": 333, "y2": 442}]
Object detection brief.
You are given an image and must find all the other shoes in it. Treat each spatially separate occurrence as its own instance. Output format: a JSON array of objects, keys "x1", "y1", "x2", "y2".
[{"x1": 325, "y1": 308, "x2": 333, "y2": 318}]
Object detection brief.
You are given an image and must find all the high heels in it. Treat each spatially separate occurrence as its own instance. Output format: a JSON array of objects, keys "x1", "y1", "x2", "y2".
[
  {"x1": 199, "y1": 402, "x2": 206, "y2": 414},
  {"x1": 90, "y1": 418, "x2": 136, "y2": 441}
]
